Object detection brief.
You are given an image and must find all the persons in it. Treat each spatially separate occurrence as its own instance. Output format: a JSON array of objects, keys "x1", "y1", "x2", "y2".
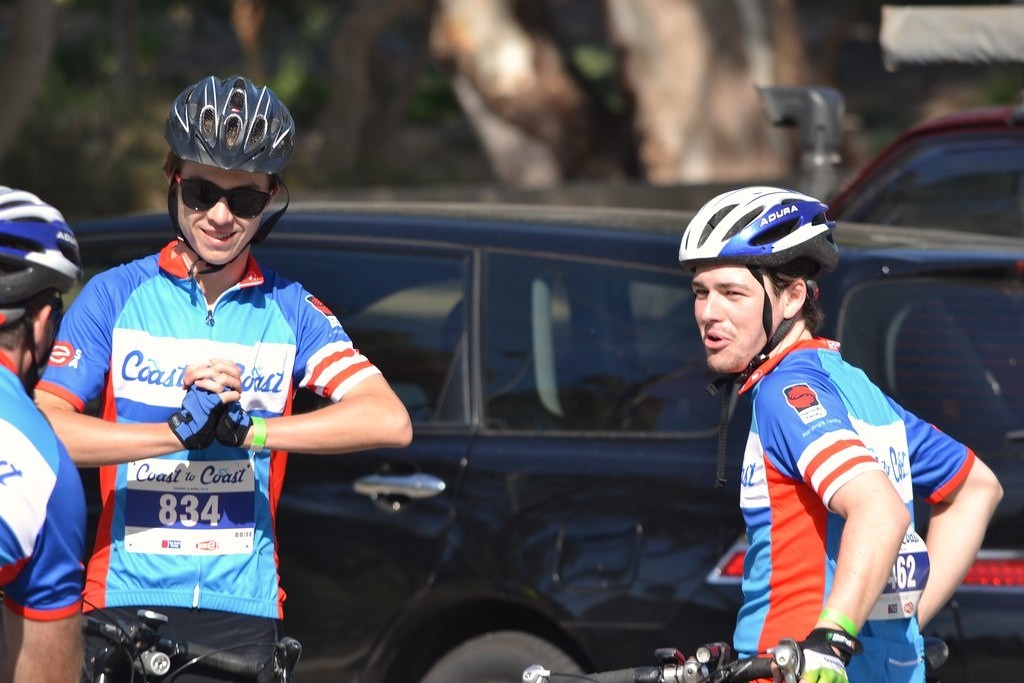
[
  {"x1": 34, "y1": 75, "x2": 413, "y2": 683},
  {"x1": 0, "y1": 185, "x2": 86, "y2": 683},
  {"x1": 678, "y1": 186, "x2": 1004, "y2": 683}
]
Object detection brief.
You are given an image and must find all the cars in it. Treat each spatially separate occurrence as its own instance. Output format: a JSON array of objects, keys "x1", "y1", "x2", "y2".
[{"x1": 64, "y1": 103, "x2": 1024, "y2": 683}]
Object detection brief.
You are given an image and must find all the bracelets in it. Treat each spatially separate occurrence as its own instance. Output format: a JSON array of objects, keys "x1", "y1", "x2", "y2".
[
  {"x1": 818, "y1": 607, "x2": 857, "y2": 638},
  {"x1": 249, "y1": 416, "x2": 267, "y2": 452}
]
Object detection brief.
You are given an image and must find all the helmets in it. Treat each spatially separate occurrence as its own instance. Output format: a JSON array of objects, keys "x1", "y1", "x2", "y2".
[
  {"x1": 679, "y1": 186, "x2": 839, "y2": 274},
  {"x1": 165, "y1": 75, "x2": 296, "y2": 176},
  {"x1": 0, "y1": 186, "x2": 81, "y2": 326}
]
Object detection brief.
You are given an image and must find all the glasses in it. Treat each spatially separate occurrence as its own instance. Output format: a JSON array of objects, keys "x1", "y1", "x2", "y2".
[{"x1": 175, "y1": 174, "x2": 273, "y2": 219}]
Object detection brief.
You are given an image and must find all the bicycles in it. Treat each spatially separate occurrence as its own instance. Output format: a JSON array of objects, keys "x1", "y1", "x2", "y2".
[
  {"x1": 522, "y1": 638, "x2": 803, "y2": 683},
  {"x1": 82, "y1": 610, "x2": 301, "y2": 683}
]
